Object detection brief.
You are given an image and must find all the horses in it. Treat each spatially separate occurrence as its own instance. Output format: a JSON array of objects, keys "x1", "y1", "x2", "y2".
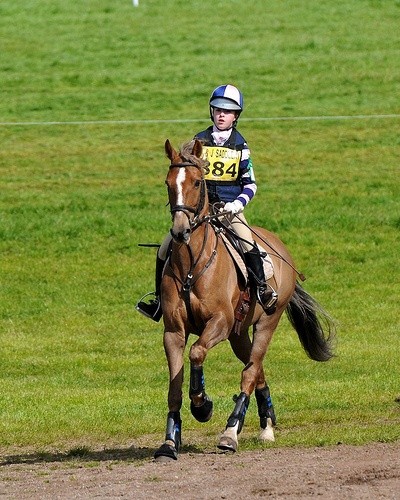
[{"x1": 153, "y1": 139, "x2": 336, "y2": 464}]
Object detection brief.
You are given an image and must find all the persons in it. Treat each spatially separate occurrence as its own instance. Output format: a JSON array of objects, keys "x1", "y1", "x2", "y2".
[{"x1": 135, "y1": 85, "x2": 278, "y2": 322}]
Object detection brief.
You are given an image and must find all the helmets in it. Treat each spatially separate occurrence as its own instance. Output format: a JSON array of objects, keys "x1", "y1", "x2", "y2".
[{"x1": 209, "y1": 84, "x2": 243, "y2": 110}]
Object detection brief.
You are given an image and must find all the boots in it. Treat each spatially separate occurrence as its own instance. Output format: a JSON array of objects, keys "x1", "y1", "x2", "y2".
[
  {"x1": 137, "y1": 247, "x2": 166, "y2": 323},
  {"x1": 237, "y1": 240, "x2": 277, "y2": 307}
]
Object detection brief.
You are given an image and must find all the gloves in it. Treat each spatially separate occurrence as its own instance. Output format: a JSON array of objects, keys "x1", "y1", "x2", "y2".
[{"x1": 223, "y1": 200, "x2": 244, "y2": 215}]
